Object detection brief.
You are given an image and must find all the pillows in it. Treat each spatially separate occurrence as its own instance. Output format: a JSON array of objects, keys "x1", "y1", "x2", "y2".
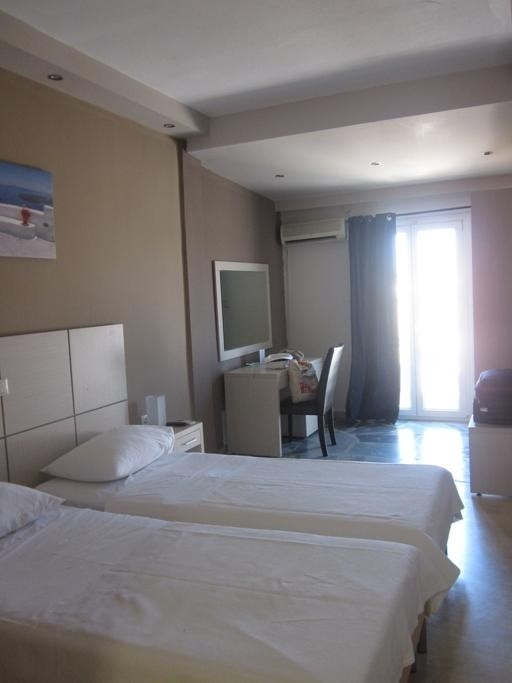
[
  {"x1": 0, "y1": 482, "x2": 65, "y2": 537},
  {"x1": 39, "y1": 424, "x2": 174, "y2": 482}
]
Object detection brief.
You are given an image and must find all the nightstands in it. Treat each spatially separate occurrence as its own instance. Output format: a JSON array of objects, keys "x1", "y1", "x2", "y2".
[{"x1": 174, "y1": 421, "x2": 205, "y2": 452}]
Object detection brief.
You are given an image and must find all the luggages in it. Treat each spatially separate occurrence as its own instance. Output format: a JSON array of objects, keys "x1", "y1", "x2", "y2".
[{"x1": 473, "y1": 368, "x2": 512, "y2": 424}]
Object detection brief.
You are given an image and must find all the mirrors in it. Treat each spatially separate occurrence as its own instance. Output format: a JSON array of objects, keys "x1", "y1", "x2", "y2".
[{"x1": 214, "y1": 260, "x2": 273, "y2": 362}]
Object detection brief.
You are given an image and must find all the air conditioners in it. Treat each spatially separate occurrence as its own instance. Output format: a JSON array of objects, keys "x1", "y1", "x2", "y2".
[{"x1": 280, "y1": 218, "x2": 345, "y2": 248}]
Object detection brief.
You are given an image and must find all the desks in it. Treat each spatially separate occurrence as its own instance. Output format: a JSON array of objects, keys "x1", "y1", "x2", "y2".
[
  {"x1": 469, "y1": 415, "x2": 511, "y2": 497},
  {"x1": 224, "y1": 357, "x2": 322, "y2": 457}
]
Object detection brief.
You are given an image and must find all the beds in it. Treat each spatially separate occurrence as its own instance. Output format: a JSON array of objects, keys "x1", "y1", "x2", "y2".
[
  {"x1": 33, "y1": 451, "x2": 464, "y2": 555},
  {"x1": 1, "y1": 508, "x2": 447, "y2": 683}
]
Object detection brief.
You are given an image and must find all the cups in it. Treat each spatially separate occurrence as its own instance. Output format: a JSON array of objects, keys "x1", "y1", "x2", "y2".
[{"x1": 145, "y1": 394, "x2": 167, "y2": 426}]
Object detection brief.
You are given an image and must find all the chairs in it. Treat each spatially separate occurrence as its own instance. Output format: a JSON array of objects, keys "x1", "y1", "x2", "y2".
[{"x1": 280, "y1": 345, "x2": 345, "y2": 456}]
